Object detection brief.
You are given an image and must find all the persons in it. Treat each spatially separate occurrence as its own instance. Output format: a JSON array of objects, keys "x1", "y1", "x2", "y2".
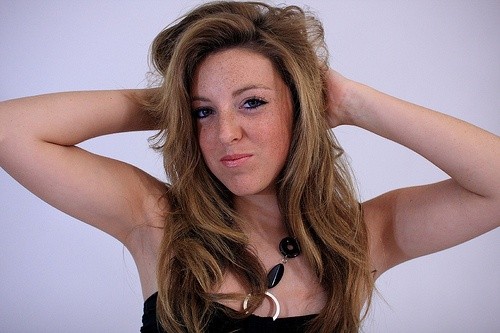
[{"x1": 1, "y1": 0, "x2": 500, "y2": 333}]
